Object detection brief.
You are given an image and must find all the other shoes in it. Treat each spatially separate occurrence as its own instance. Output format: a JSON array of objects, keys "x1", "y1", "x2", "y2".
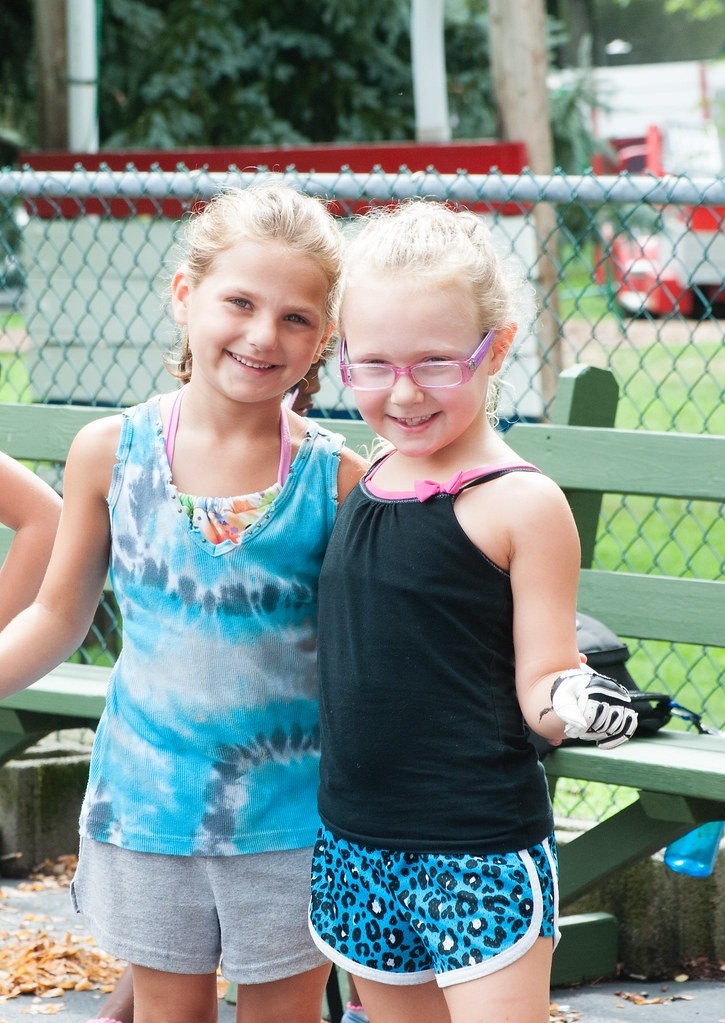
[{"x1": 341, "y1": 1010, "x2": 369, "y2": 1023}]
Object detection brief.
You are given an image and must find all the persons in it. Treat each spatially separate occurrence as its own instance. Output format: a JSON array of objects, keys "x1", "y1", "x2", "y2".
[
  {"x1": 2, "y1": 178, "x2": 588, "y2": 1023},
  {"x1": 308, "y1": 201, "x2": 637, "y2": 1023},
  {"x1": 0, "y1": 445, "x2": 64, "y2": 638}
]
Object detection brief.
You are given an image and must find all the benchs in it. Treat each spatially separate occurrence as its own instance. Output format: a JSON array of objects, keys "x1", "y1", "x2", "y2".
[{"x1": 0, "y1": 367, "x2": 725, "y2": 1023}]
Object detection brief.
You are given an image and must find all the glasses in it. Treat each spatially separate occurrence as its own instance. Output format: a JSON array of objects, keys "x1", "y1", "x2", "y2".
[{"x1": 340, "y1": 325, "x2": 498, "y2": 392}]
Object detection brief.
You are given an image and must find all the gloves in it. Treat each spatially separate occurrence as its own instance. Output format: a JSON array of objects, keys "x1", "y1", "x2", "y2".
[{"x1": 550, "y1": 662, "x2": 638, "y2": 750}]
